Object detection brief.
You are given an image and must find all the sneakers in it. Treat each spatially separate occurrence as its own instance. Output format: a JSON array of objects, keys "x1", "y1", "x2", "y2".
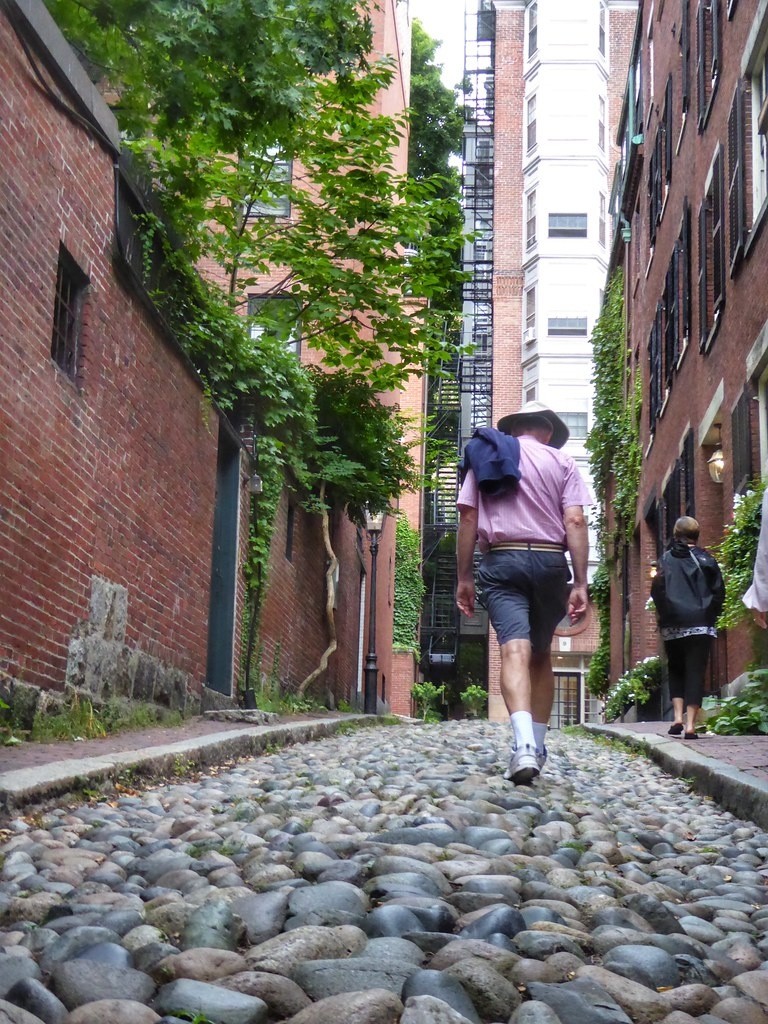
[
  {"x1": 535, "y1": 744, "x2": 547, "y2": 772},
  {"x1": 503, "y1": 744, "x2": 540, "y2": 783}
]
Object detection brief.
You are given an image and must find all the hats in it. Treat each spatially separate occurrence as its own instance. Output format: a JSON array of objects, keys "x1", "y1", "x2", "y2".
[{"x1": 497, "y1": 401, "x2": 570, "y2": 449}]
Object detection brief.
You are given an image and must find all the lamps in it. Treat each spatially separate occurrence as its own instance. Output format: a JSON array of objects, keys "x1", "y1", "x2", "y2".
[{"x1": 707, "y1": 423, "x2": 726, "y2": 483}]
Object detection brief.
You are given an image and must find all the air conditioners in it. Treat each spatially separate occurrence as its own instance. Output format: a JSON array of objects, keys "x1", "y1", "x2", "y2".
[{"x1": 522, "y1": 328, "x2": 535, "y2": 343}]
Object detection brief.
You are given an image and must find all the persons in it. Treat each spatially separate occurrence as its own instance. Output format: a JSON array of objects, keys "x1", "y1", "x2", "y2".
[
  {"x1": 742, "y1": 488, "x2": 768, "y2": 628},
  {"x1": 456, "y1": 400, "x2": 594, "y2": 781},
  {"x1": 650, "y1": 517, "x2": 726, "y2": 739}
]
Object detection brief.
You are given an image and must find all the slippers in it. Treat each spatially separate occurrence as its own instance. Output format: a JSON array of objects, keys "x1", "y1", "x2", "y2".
[
  {"x1": 685, "y1": 730, "x2": 698, "y2": 739},
  {"x1": 668, "y1": 722, "x2": 683, "y2": 737}
]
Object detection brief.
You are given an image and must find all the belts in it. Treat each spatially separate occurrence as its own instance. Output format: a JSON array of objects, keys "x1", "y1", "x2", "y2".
[{"x1": 488, "y1": 541, "x2": 564, "y2": 553}]
